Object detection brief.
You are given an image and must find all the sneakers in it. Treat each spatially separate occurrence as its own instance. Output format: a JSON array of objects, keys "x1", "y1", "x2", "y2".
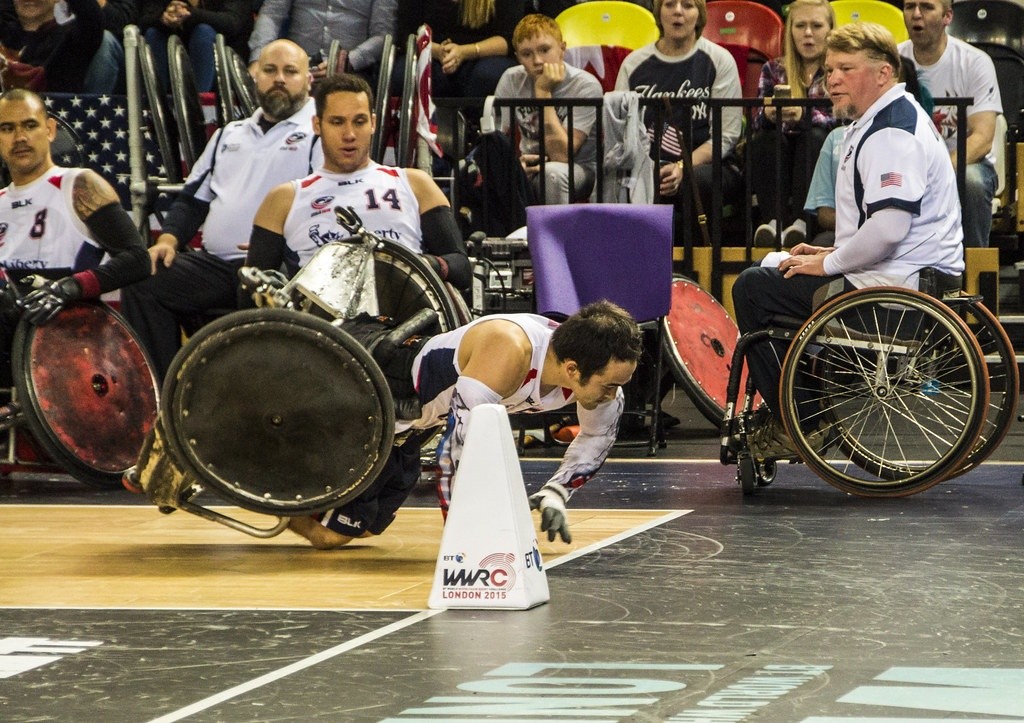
[{"x1": 733, "y1": 412, "x2": 836, "y2": 464}]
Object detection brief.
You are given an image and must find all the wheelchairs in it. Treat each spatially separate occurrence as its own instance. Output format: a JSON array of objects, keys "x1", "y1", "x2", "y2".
[
  {"x1": 644, "y1": 272, "x2": 764, "y2": 427},
  {"x1": 123, "y1": 207, "x2": 472, "y2": 538},
  {"x1": 0, "y1": 260, "x2": 161, "y2": 491},
  {"x1": 720, "y1": 266, "x2": 1018, "y2": 497}
]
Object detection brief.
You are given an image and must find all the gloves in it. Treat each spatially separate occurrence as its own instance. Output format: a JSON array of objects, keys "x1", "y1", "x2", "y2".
[
  {"x1": 528, "y1": 488, "x2": 571, "y2": 543},
  {"x1": 11, "y1": 274, "x2": 79, "y2": 327}
]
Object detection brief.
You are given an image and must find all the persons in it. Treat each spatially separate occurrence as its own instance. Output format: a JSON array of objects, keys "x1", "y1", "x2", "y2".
[
  {"x1": 1, "y1": 39, "x2": 473, "y2": 425},
  {"x1": 731, "y1": 21, "x2": 966, "y2": 459},
  {"x1": 0, "y1": 0, "x2": 1006, "y2": 247},
  {"x1": 287, "y1": 299, "x2": 643, "y2": 550}
]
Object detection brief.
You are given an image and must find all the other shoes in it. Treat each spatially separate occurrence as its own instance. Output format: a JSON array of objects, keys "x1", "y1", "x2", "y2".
[
  {"x1": 782, "y1": 225, "x2": 806, "y2": 247},
  {"x1": 754, "y1": 224, "x2": 776, "y2": 248}
]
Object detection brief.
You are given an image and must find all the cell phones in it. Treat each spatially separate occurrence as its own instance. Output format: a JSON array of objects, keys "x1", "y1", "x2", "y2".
[{"x1": 774, "y1": 85, "x2": 792, "y2": 98}]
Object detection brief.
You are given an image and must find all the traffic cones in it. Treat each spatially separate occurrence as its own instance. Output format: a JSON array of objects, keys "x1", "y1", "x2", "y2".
[{"x1": 427, "y1": 403, "x2": 550, "y2": 611}]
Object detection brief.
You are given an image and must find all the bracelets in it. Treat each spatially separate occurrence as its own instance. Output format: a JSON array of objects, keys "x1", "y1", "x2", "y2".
[{"x1": 474, "y1": 43, "x2": 480, "y2": 58}]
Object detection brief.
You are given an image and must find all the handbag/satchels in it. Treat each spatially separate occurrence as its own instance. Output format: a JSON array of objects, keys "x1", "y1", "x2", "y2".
[{"x1": 474, "y1": 129, "x2": 538, "y2": 237}]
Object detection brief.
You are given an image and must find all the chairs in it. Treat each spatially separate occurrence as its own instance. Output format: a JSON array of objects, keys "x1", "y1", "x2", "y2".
[{"x1": 517, "y1": 0, "x2": 1024, "y2": 452}]
